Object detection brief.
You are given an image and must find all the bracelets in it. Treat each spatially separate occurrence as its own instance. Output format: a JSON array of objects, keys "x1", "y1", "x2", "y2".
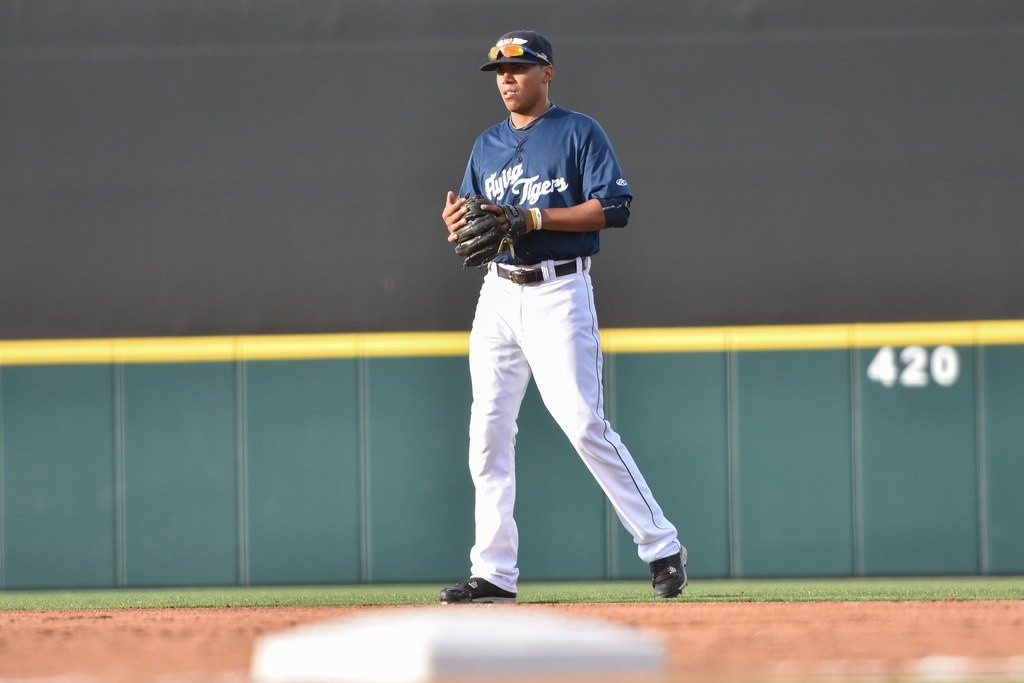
[{"x1": 527, "y1": 207, "x2": 543, "y2": 230}]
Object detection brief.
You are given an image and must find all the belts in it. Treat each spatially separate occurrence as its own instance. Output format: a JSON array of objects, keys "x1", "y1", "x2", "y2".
[{"x1": 488, "y1": 258, "x2": 587, "y2": 285}]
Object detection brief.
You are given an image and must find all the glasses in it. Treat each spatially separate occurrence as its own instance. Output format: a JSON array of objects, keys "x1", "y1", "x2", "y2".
[{"x1": 488, "y1": 43, "x2": 552, "y2": 64}]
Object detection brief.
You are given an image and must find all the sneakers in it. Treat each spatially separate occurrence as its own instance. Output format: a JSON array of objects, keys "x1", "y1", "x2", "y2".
[
  {"x1": 649, "y1": 544, "x2": 688, "y2": 598},
  {"x1": 439, "y1": 578, "x2": 518, "y2": 605}
]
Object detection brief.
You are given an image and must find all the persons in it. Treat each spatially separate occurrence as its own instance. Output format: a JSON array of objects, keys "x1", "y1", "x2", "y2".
[{"x1": 437, "y1": 26, "x2": 688, "y2": 605}]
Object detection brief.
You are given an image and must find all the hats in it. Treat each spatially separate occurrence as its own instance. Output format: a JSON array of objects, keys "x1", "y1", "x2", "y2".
[{"x1": 480, "y1": 30, "x2": 553, "y2": 72}]
